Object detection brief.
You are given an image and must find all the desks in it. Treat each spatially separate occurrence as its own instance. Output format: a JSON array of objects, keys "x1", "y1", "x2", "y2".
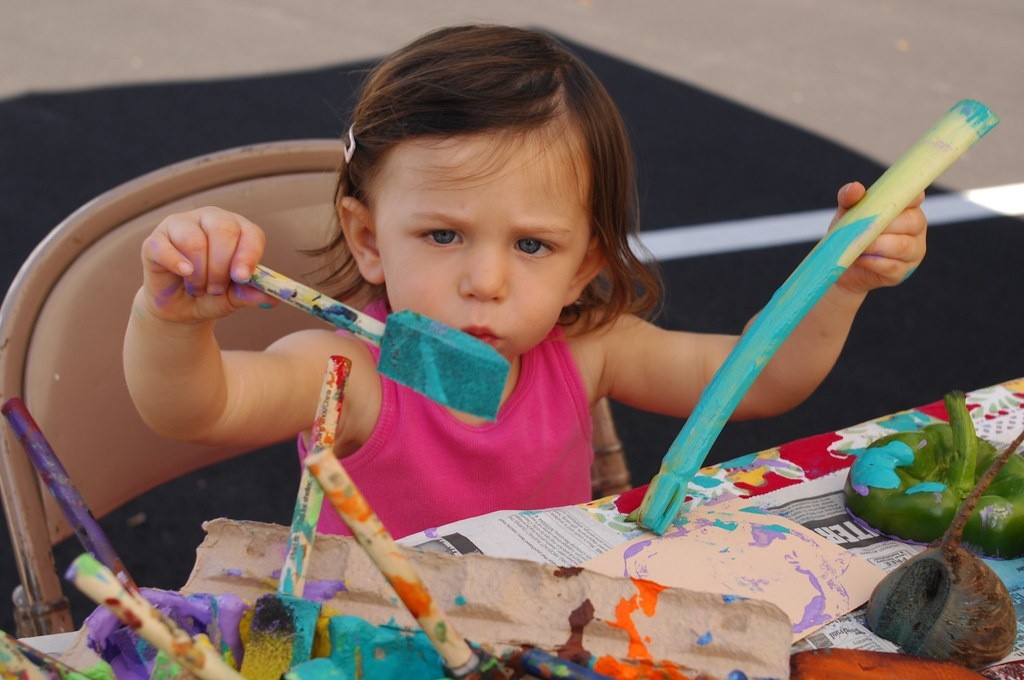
[{"x1": 20, "y1": 374, "x2": 1024, "y2": 680}]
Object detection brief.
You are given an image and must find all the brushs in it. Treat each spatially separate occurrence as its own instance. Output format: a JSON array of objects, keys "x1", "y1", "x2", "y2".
[{"x1": 234, "y1": 262, "x2": 509, "y2": 423}]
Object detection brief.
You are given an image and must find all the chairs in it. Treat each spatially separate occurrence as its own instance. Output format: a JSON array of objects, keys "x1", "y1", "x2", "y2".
[{"x1": 0, "y1": 136, "x2": 636, "y2": 639}]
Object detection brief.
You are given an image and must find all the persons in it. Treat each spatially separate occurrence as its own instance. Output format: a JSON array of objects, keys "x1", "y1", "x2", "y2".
[{"x1": 122, "y1": 25, "x2": 927, "y2": 546}]
